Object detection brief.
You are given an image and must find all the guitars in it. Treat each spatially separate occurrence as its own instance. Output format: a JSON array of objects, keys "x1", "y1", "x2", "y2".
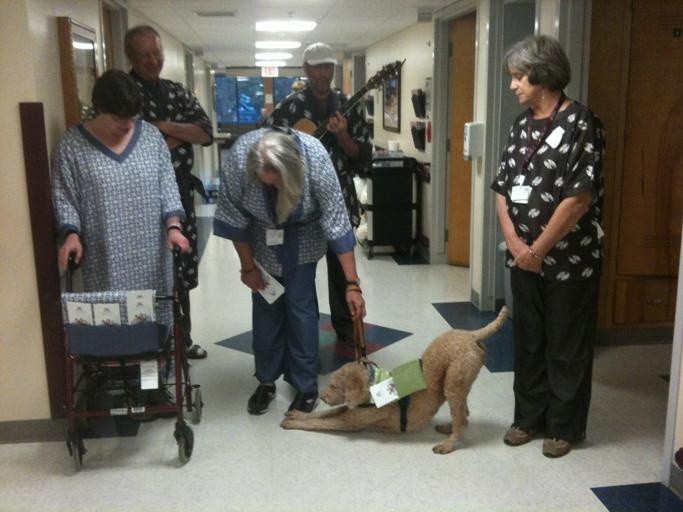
[{"x1": 292, "y1": 60, "x2": 405, "y2": 145}]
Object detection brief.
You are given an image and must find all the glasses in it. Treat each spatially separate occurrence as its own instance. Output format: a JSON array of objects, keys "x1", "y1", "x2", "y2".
[{"x1": 109, "y1": 113, "x2": 140, "y2": 122}]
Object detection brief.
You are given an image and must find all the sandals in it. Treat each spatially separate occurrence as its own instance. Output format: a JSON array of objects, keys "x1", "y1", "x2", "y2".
[
  {"x1": 287, "y1": 390, "x2": 318, "y2": 414},
  {"x1": 247, "y1": 382, "x2": 275, "y2": 414}
]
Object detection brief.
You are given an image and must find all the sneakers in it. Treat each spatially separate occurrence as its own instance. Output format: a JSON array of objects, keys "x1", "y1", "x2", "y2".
[
  {"x1": 543, "y1": 436, "x2": 571, "y2": 457},
  {"x1": 336, "y1": 336, "x2": 361, "y2": 360},
  {"x1": 119, "y1": 395, "x2": 155, "y2": 421},
  {"x1": 504, "y1": 422, "x2": 545, "y2": 445},
  {"x1": 141, "y1": 389, "x2": 177, "y2": 418}
]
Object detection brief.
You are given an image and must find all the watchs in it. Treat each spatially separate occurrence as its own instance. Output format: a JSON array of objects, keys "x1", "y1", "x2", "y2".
[
  {"x1": 345, "y1": 278, "x2": 360, "y2": 286},
  {"x1": 167, "y1": 223, "x2": 184, "y2": 232}
]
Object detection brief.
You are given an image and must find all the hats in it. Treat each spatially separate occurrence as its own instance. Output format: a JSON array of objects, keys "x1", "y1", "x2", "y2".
[{"x1": 302, "y1": 42, "x2": 337, "y2": 66}]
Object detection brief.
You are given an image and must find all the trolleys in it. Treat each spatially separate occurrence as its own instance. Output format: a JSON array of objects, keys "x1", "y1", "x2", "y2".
[{"x1": 63, "y1": 245, "x2": 204, "y2": 472}]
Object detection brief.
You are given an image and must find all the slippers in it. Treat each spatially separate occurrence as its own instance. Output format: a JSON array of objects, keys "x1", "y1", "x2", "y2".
[{"x1": 184, "y1": 342, "x2": 207, "y2": 359}]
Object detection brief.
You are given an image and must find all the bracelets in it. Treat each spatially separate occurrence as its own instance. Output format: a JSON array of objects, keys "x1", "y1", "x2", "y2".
[
  {"x1": 240, "y1": 265, "x2": 256, "y2": 273},
  {"x1": 529, "y1": 246, "x2": 544, "y2": 261}
]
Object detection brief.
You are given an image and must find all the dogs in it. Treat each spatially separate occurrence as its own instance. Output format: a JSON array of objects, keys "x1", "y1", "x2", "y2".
[{"x1": 279, "y1": 304, "x2": 508, "y2": 455}]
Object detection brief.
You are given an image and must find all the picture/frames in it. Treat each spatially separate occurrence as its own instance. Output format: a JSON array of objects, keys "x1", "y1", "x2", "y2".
[{"x1": 382, "y1": 71, "x2": 401, "y2": 133}]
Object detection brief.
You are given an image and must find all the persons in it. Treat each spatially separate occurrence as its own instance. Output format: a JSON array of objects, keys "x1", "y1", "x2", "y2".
[
  {"x1": 123, "y1": 24, "x2": 213, "y2": 358},
  {"x1": 213, "y1": 124, "x2": 366, "y2": 415},
  {"x1": 48, "y1": 68, "x2": 192, "y2": 418},
  {"x1": 490, "y1": 34, "x2": 606, "y2": 458},
  {"x1": 263, "y1": 42, "x2": 372, "y2": 346}
]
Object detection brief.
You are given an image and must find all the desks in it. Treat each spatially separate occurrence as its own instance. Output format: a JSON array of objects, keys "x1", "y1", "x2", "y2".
[{"x1": 359, "y1": 150, "x2": 431, "y2": 259}]
